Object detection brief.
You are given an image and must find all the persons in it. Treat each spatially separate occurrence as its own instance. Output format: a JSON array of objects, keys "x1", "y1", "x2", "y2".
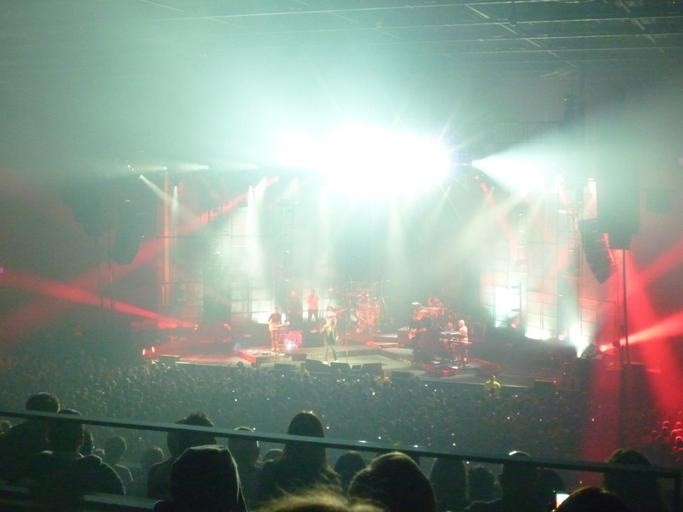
[
  {"x1": 2, "y1": 327, "x2": 683, "y2": 511},
  {"x1": 264, "y1": 285, "x2": 471, "y2": 372}
]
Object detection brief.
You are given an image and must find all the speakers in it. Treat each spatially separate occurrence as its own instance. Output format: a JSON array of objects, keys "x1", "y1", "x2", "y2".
[
  {"x1": 113, "y1": 202, "x2": 148, "y2": 265},
  {"x1": 579, "y1": 218, "x2": 616, "y2": 283},
  {"x1": 159, "y1": 354, "x2": 180, "y2": 367},
  {"x1": 255, "y1": 348, "x2": 416, "y2": 379}
]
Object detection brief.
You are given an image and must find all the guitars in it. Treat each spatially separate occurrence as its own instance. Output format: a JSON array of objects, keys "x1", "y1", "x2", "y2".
[{"x1": 269, "y1": 322, "x2": 290, "y2": 332}]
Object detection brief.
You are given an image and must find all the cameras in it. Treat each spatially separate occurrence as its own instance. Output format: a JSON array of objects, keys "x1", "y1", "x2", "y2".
[{"x1": 552, "y1": 490, "x2": 571, "y2": 506}]
